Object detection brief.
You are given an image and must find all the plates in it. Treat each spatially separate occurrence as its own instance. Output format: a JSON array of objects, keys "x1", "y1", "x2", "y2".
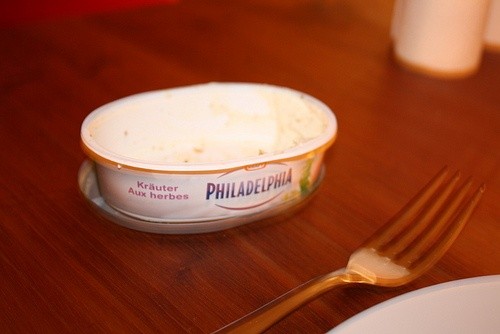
[{"x1": 326, "y1": 275, "x2": 499, "y2": 333}]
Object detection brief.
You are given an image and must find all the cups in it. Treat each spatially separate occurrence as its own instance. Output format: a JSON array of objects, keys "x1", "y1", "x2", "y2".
[{"x1": 390, "y1": 1, "x2": 500, "y2": 78}]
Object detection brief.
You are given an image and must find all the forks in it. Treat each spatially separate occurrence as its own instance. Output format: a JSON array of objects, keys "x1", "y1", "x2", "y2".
[{"x1": 211, "y1": 165, "x2": 487, "y2": 334}]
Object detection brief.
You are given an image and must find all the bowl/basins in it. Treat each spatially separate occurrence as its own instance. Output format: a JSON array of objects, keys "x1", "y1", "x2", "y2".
[{"x1": 78, "y1": 81, "x2": 337, "y2": 225}]
